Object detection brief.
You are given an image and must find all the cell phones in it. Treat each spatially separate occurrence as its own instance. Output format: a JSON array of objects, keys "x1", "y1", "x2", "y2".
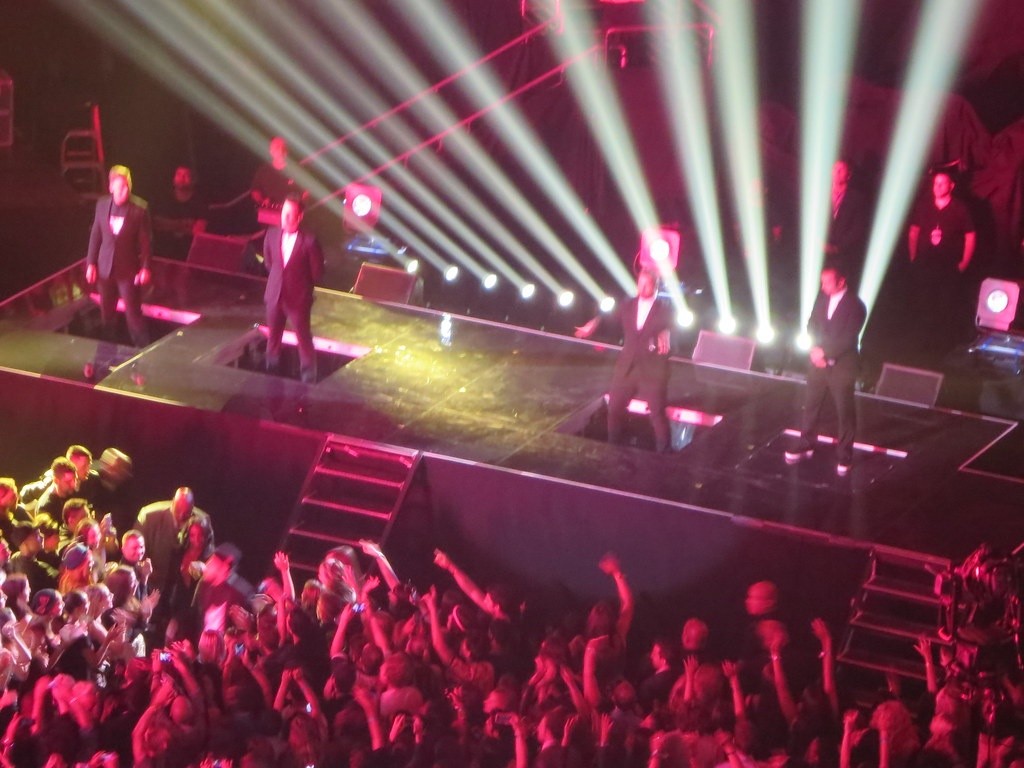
[
  {"x1": 410, "y1": 587, "x2": 417, "y2": 600},
  {"x1": 234, "y1": 643, "x2": 243, "y2": 658}
]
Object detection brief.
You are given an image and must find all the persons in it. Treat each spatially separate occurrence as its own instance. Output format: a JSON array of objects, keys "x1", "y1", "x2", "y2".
[
  {"x1": 908, "y1": 169, "x2": 977, "y2": 284},
  {"x1": 784, "y1": 267, "x2": 866, "y2": 472},
  {"x1": 86, "y1": 164, "x2": 152, "y2": 345},
  {"x1": 251, "y1": 135, "x2": 308, "y2": 217},
  {"x1": 573, "y1": 268, "x2": 674, "y2": 453},
  {"x1": 824, "y1": 157, "x2": 867, "y2": 265},
  {"x1": 261, "y1": 196, "x2": 325, "y2": 383},
  {"x1": 0, "y1": 445, "x2": 1024, "y2": 768},
  {"x1": 152, "y1": 160, "x2": 208, "y2": 258},
  {"x1": 732, "y1": 184, "x2": 782, "y2": 260}
]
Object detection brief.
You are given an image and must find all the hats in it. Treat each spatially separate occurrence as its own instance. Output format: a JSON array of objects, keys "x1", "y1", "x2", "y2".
[
  {"x1": 31, "y1": 589, "x2": 55, "y2": 616},
  {"x1": 1, "y1": 573, "x2": 26, "y2": 596},
  {"x1": 64, "y1": 544, "x2": 89, "y2": 570},
  {"x1": 10, "y1": 522, "x2": 41, "y2": 548}
]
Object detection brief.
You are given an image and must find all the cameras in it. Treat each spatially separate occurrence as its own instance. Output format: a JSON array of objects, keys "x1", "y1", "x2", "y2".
[
  {"x1": 351, "y1": 601, "x2": 365, "y2": 613},
  {"x1": 160, "y1": 651, "x2": 172, "y2": 662}
]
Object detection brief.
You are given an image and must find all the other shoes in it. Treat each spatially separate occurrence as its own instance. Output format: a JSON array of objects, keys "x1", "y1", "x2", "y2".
[
  {"x1": 837, "y1": 461, "x2": 851, "y2": 471},
  {"x1": 785, "y1": 446, "x2": 814, "y2": 459}
]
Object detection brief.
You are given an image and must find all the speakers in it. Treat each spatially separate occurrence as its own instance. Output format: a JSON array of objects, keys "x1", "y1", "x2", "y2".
[
  {"x1": 353, "y1": 264, "x2": 424, "y2": 309},
  {"x1": 186, "y1": 232, "x2": 257, "y2": 274},
  {"x1": 691, "y1": 331, "x2": 756, "y2": 371},
  {"x1": 875, "y1": 363, "x2": 943, "y2": 408}
]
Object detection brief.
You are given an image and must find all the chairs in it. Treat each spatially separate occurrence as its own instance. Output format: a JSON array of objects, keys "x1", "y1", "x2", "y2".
[{"x1": 61, "y1": 102, "x2": 107, "y2": 193}]
